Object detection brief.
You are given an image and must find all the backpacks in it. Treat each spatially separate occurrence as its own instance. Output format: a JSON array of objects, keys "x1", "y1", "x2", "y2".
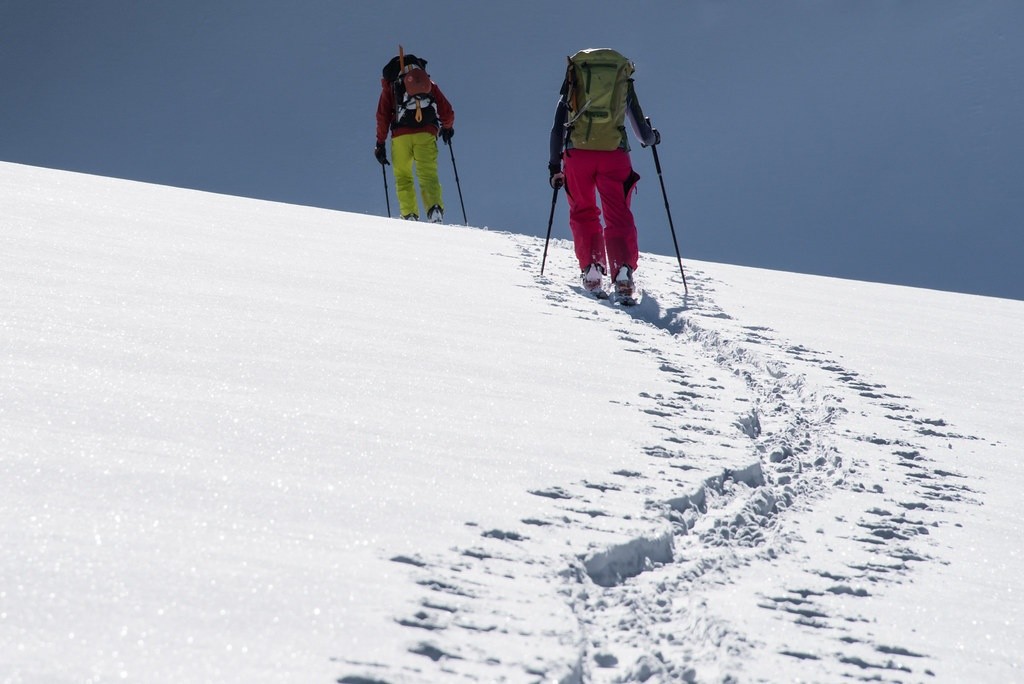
[
  {"x1": 383, "y1": 54, "x2": 441, "y2": 127},
  {"x1": 564, "y1": 48, "x2": 635, "y2": 151}
]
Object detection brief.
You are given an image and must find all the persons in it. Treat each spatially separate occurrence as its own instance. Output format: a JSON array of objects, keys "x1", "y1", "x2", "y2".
[
  {"x1": 548, "y1": 90, "x2": 661, "y2": 296},
  {"x1": 374, "y1": 64, "x2": 454, "y2": 223}
]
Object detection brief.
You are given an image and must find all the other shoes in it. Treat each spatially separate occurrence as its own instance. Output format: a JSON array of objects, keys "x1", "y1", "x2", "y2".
[
  {"x1": 614, "y1": 264, "x2": 636, "y2": 305},
  {"x1": 427, "y1": 204, "x2": 443, "y2": 224},
  {"x1": 581, "y1": 265, "x2": 607, "y2": 297},
  {"x1": 400, "y1": 214, "x2": 419, "y2": 221}
]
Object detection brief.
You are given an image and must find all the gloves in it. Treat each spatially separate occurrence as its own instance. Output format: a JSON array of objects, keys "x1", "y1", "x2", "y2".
[
  {"x1": 439, "y1": 127, "x2": 454, "y2": 145},
  {"x1": 374, "y1": 142, "x2": 390, "y2": 165},
  {"x1": 551, "y1": 172, "x2": 565, "y2": 189},
  {"x1": 652, "y1": 128, "x2": 661, "y2": 145}
]
